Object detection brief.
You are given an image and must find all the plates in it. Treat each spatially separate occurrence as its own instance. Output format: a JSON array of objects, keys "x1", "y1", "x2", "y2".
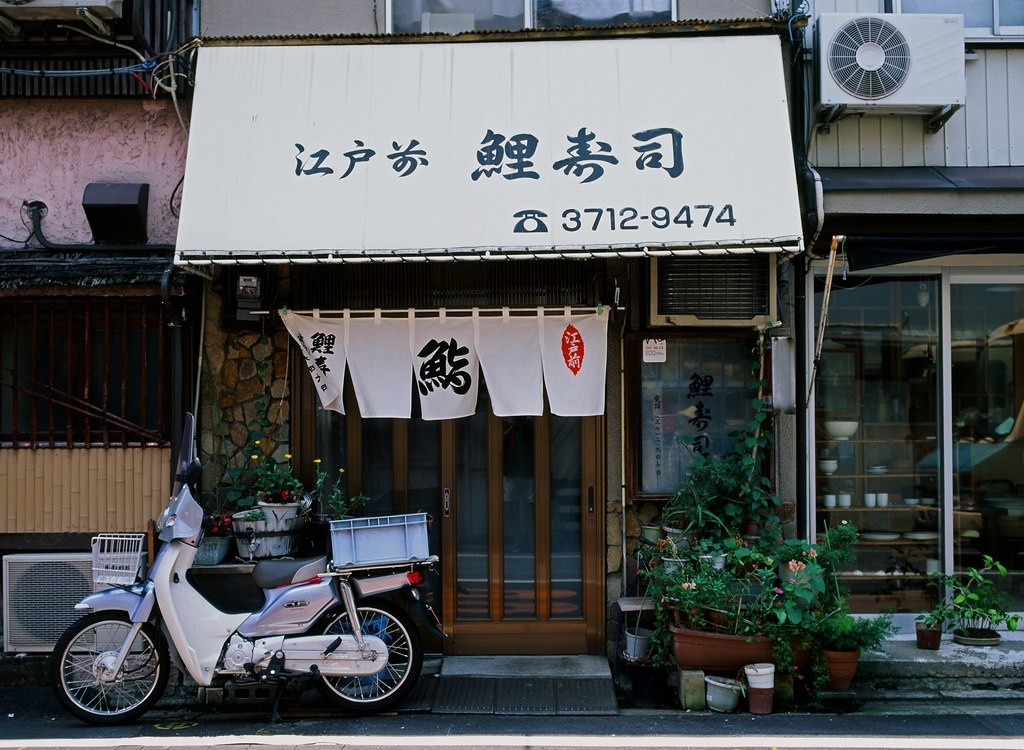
[
  {"x1": 904, "y1": 532, "x2": 941, "y2": 541},
  {"x1": 867, "y1": 466, "x2": 888, "y2": 475},
  {"x1": 860, "y1": 533, "x2": 901, "y2": 540}
]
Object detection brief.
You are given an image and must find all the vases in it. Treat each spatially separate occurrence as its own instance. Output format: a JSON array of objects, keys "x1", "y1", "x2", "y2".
[
  {"x1": 641, "y1": 525, "x2": 662, "y2": 552},
  {"x1": 193, "y1": 538, "x2": 229, "y2": 564},
  {"x1": 625, "y1": 627, "x2": 654, "y2": 660},
  {"x1": 744, "y1": 663, "x2": 777, "y2": 715},
  {"x1": 742, "y1": 535, "x2": 761, "y2": 552},
  {"x1": 661, "y1": 555, "x2": 690, "y2": 575},
  {"x1": 706, "y1": 676, "x2": 745, "y2": 713},
  {"x1": 312, "y1": 521, "x2": 333, "y2": 563},
  {"x1": 232, "y1": 501, "x2": 303, "y2": 559},
  {"x1": 821, "y1": 648, "x2": 861, "y2": 683},
  {"x1": 662, "y1": 520, "x2": 695, "y2": 550},
  {"x1": 667, "y1": 620, "x2": 813, "y2": 671},
  {"x1": 698, "y1": 554, "x2": 728, "y2": 573}
]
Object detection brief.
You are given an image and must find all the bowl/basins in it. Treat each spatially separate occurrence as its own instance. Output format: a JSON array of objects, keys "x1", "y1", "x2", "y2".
[
  {"x1": 823, "y1": 421, "x2": 859, "y2": 441},
  {"x1": 904, "y1": 499, "x2": 919, "y2": 507},
  {"x1": 921, "y1": 498, "x2": 935, "y2": 505},
  {"x1": 819, "y1": 460, "x2": 838, "y2": 476}
]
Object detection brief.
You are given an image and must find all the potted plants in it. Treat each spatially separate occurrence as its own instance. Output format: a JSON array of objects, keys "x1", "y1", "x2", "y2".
[{"x1": 915, "y1": 554, "x2": 1024, "y2": 650}]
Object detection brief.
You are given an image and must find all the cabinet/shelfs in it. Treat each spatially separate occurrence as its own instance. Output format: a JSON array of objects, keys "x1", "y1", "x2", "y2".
[{"x1": 818, "y1": 303, "x2": 1021, "y2": 612}]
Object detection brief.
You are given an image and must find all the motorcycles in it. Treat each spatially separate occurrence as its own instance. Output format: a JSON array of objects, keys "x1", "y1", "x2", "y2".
[{"x1": 50, "y1": 412, "x2": 456, "y2": 727}]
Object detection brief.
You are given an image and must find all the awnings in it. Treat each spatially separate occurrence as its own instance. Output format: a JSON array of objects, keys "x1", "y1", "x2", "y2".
[
  {"x1": 172, "y1": 33, "x2": 808, "y2": 282},
  {"x1": 806, "y1": 210, "x2": 1024, "y2": 398}
]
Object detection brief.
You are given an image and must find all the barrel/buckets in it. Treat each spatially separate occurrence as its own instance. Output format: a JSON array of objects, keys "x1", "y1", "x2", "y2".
[
  {"x1": 747, "y1": 687, "x2": 774, "y2": 715},
  {"x1": 744, "y1": 663, "x2": 775, "y2": 688},
  {"x1": 625, "y1": 628, "x2": 652, "y2": 658}
]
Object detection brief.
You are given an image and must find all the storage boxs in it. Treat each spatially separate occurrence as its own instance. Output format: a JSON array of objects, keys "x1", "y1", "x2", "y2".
[{"x1": 329, "y1": 513, "x2": 429, "y2": 568}]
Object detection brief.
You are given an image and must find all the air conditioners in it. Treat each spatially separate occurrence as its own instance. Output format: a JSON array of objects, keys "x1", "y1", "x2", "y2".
[
  {"x1": 2, "y1": 553, "x2": 148, "y2": 653},
  {"x1": 0, "y1": 0, "x2": 123, "y2": 36},
  {"x1": 810, "y1": 14, "x2": 966, "y2": 115},
  {"x1": 650, "y1": 247, "x2": 779, "y2": 330}
]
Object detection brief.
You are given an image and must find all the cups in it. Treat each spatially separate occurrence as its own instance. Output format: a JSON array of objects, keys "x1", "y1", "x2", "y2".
[
  {"x1": 824, "y1": 495, "x2": 836, "y2": 509},
  {"x1": 877, "y1": 493, "x2": 888, "y2": 507},
  {"x1": 864, "y1": 493, "x2": 875, "y2": 507},
  {"x1": 838, "y1": 495, "x2": 851, "y2": 509}
]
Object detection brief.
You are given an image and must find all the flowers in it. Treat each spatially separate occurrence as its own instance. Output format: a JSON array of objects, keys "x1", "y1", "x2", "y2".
[
  {"x1": 202, "y1": 509, "x2": 234, "y2": 538},
  {"x1": 189, "y1": 319, "x2": 372, "y2": 535},
  {"x1": 643, "y1": 405, "x2": 901, "y2": 684}
]
